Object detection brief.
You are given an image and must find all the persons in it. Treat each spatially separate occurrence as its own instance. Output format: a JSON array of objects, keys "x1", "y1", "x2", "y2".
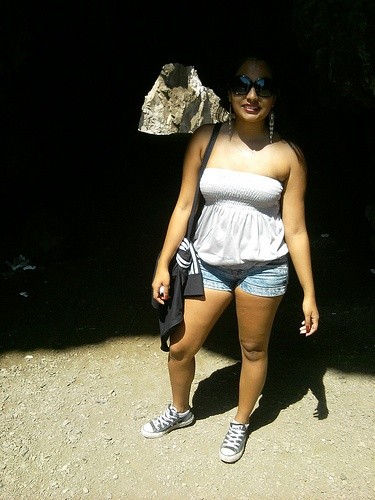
[{"x1": 140, "y1": 44, "x2": 319, "y2": 465}]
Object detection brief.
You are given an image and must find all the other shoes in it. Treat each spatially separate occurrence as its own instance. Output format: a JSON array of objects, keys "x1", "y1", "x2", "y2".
[
  {"x1": 140, "y1": 404, "x2": 195, "y2": 439},
  {"x1": 219, "y1": 420, "x2": 249, "y2": 464}
]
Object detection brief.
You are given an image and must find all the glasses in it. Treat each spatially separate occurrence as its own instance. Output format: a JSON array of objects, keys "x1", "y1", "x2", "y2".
[{"x1": 230, "y1": 74, "x2": 274, "y2": 97}]
